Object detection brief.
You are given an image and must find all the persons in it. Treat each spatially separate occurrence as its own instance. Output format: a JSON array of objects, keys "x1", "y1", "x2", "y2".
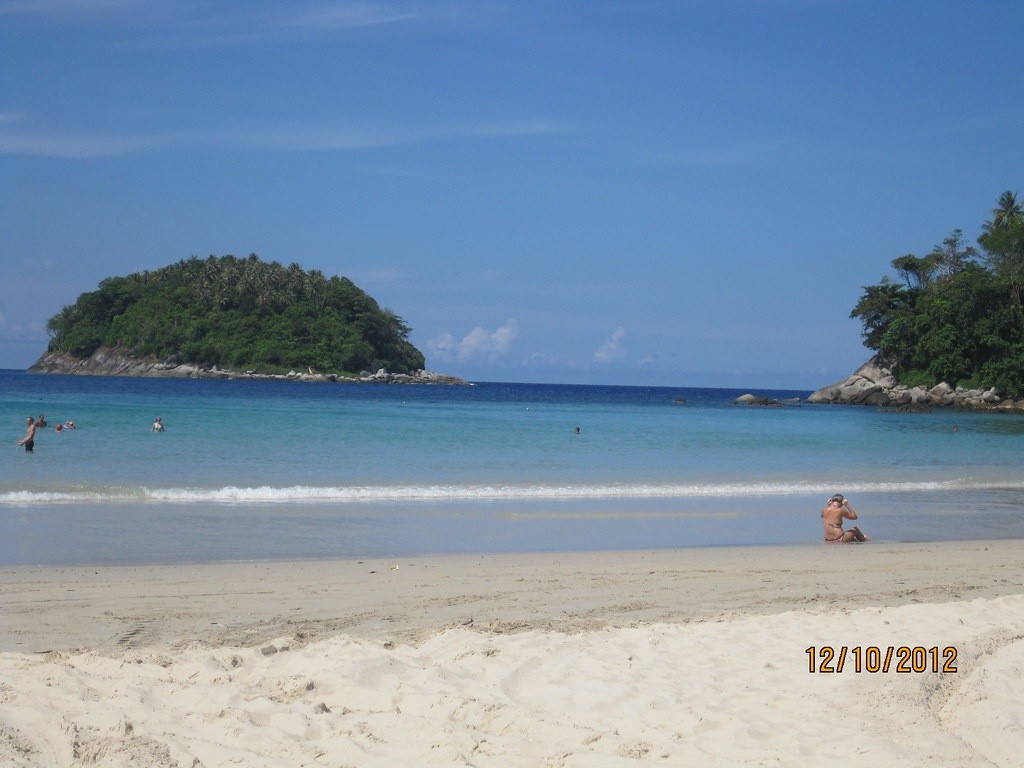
[
  {"x1": 55, "y1": 424, "x2": 63, "y2": 432},
  {"x1": 576, "y1": 427, "x2": 580, "y2": 433},
  {"x1": 151, "y1": 416, "x2": 166, "y2": 432},
  {"x1": 820, "y1": 494, "x2": 872, "y2": 544},
  {"x1": 17, "y1": 417, "x2": 36, "y2": 453},
  {"x1": 66, "y1": 420, "x2": 77, "y2": 430},
  {"x1": 35, "y1": 414, "x2": 47, "y2": 428}
]
